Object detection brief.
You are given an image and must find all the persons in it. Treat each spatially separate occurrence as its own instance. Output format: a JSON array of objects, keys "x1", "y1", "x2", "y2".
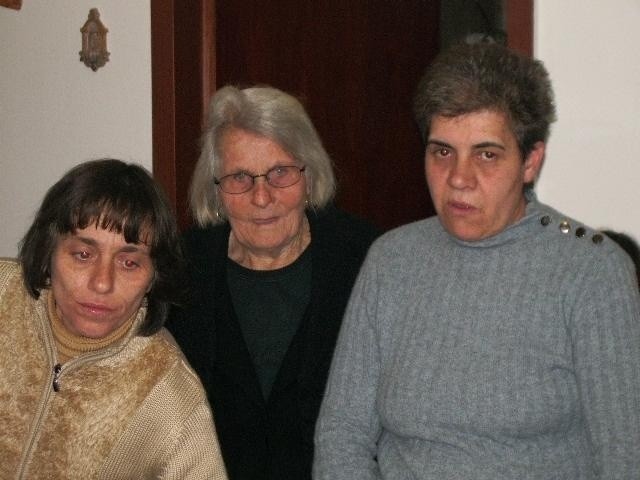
[
  {"x1": 162, "y1": 84, "x2": 386, "y2": 479},
  {"x1": 308, "y1": 31, "x2": 639, "y2": 478},
  {"x1": 0, "y1": 157, "x2": 229, "y2": 480}
]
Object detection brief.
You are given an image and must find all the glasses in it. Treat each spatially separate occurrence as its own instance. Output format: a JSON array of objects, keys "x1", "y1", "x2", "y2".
[{"x1": 211, "y1": 164, "x2": 306, "y2": 196}]
